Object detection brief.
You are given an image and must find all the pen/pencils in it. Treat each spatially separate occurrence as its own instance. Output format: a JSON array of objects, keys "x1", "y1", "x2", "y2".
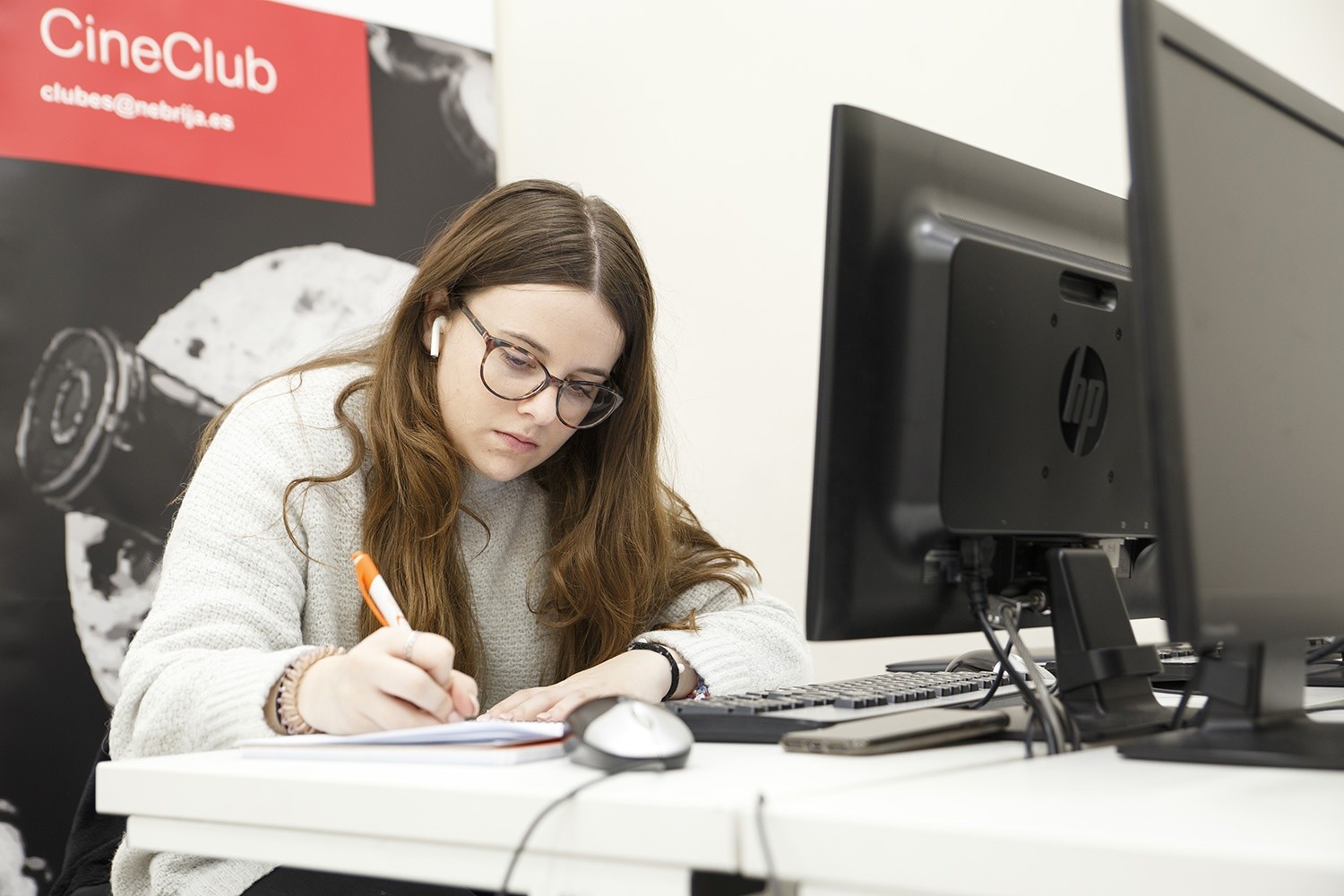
[{"x1": 350, "y1": 549, "x2": 468, "y2": 722}]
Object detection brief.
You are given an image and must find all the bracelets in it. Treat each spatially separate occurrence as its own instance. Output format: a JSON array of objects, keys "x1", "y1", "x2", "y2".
[
  {"x1": 276, "y1": 643, "x2": 346, "y2": 735},
  {"x1": 627, "y1": 638, "x2": 685, "y2": 701}
]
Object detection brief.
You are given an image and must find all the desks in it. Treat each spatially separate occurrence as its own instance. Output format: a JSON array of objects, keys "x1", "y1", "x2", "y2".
[{"x1": 95, "y1": 683, "x2": 1344, "y2": 896}]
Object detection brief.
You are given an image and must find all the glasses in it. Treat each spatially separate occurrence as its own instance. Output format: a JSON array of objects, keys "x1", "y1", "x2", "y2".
[{"x1": 455, "y1": 295, "x2": 623, "y2": 430}]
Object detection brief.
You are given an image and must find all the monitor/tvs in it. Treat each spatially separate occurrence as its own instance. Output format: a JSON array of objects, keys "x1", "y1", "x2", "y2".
[
  {"x1": 1122, "y1": 0, "x2": 1344, "y2": 771},
  {"x1": 807, "y1": 105, "x2": 1202, "y2": 746}
]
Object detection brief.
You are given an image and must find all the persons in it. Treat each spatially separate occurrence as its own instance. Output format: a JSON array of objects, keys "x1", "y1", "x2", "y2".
[{"x1": 110, "y1": 180, "x2": 812, "y2": 896}]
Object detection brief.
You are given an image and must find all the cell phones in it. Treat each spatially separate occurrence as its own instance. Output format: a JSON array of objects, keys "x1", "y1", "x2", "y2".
[{"x1": 783, "y1": 707, "x2": 1009, "y2": 759}]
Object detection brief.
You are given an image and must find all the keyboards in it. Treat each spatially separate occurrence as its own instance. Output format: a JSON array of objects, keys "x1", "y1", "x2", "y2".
[
  {"x1": 644, "y1": 669, "x2": 1048, "y2": 743},
  {"x1": 886, "y1": 642, "x2": 1201, "y2": 686}
]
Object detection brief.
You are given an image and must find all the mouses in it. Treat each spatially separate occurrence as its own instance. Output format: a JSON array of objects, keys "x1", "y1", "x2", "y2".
[
  {"x1": 944, "y1": 648, "x2": 1058, "y2": 685},
  {"x1": 559, "y1": 695, "x2": 695, "y2": 772}
]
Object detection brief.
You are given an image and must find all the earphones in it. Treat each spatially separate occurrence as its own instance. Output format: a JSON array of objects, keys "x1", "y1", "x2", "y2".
[{"x1": 430, "y1": 313, "x2": 447, "y2": 360}]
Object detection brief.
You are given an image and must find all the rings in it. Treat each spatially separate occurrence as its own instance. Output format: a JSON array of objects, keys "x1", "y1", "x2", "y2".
[{"x1": 403, "y1": 631, "x2": 419, "y2": 662}]
